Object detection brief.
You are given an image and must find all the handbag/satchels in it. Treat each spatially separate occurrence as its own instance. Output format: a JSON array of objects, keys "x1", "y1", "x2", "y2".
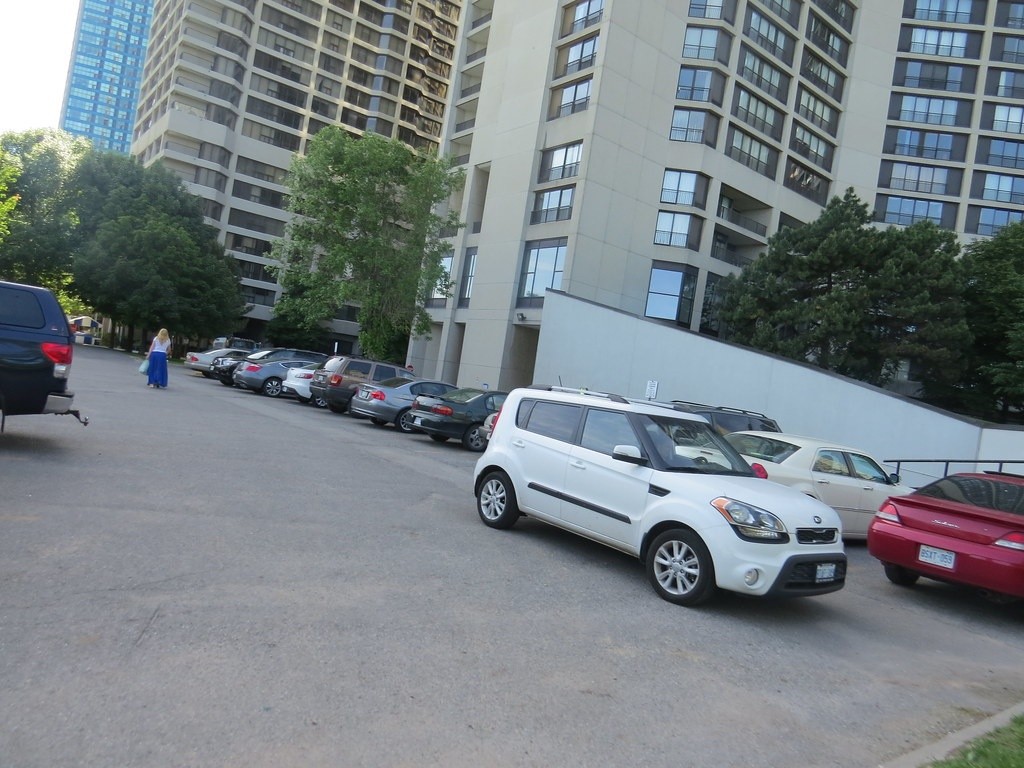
[{"x1": 139, "y1": 359, "x2": 149, "y2": 375}]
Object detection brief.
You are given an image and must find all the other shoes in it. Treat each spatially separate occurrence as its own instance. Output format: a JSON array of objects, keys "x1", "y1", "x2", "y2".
[{"x1": 148, "y1": 384, "x2": 159, "y2": 388}]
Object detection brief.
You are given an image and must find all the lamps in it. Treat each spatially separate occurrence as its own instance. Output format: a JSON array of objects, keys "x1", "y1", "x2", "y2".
[{"x1": 516, "y1": 312, "x2": 527, "y2": 321}]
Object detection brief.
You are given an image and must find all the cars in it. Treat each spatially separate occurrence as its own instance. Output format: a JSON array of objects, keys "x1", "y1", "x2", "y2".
[
  {"x1": 478, "y1": 412, "x2": 499, "y2": 446},
  {"x1": 184, "y1": 348, "x2": 254, "y2": 378},
  {"x1": 671, "y1": 400, "x2": 790, "y2": 454},
  {"x1": 232, "y1": 358, "x2": 317, "y2": 397},
  {"x1": 868, "y1": 472, "x2": 1024, "y2": 601},
  {"x1": 281, "y1": 363, "x2": 327, "y2": 408},
  {"x1": 404, "y1": 389, "x2": 512, "y2": 452},
  {"x1": 213, "y1": 338, "x2": 257, "y2": 349},
  {"x1": 208, "y1": 347, "x2": 329, "y2": 386},
  {"x1": 676, "y1": 431, "x2": 918, "y2": 539},
  {"x1": 351, "y1": 376, "x2": 461, "y2": 433},
  {"x1": 0, "y1": 281, "x2": 89, "y2": 431}
]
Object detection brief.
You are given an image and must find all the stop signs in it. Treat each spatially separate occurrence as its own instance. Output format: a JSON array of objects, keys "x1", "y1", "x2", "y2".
[{"x1": 407, "y1": 365, "x2": 414, "y2": 371}]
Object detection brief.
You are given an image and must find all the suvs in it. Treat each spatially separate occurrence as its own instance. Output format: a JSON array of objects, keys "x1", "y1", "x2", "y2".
[
  {"x1": 309, "y1": 354, "x2": 417, "y2": 419},
  {"x1": 474, "y1": 385, "x2": 847, "y2": 605}
]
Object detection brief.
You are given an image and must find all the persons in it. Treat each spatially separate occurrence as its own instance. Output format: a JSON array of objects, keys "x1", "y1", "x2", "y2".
[{"x1": 146, "y1": 329, "x2": 171, "y2": 388}]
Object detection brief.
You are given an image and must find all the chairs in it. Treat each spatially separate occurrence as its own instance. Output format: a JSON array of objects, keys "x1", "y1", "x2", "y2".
[{"x1": 818, "y1": 454, "x2": 834, "y2": 473}]
